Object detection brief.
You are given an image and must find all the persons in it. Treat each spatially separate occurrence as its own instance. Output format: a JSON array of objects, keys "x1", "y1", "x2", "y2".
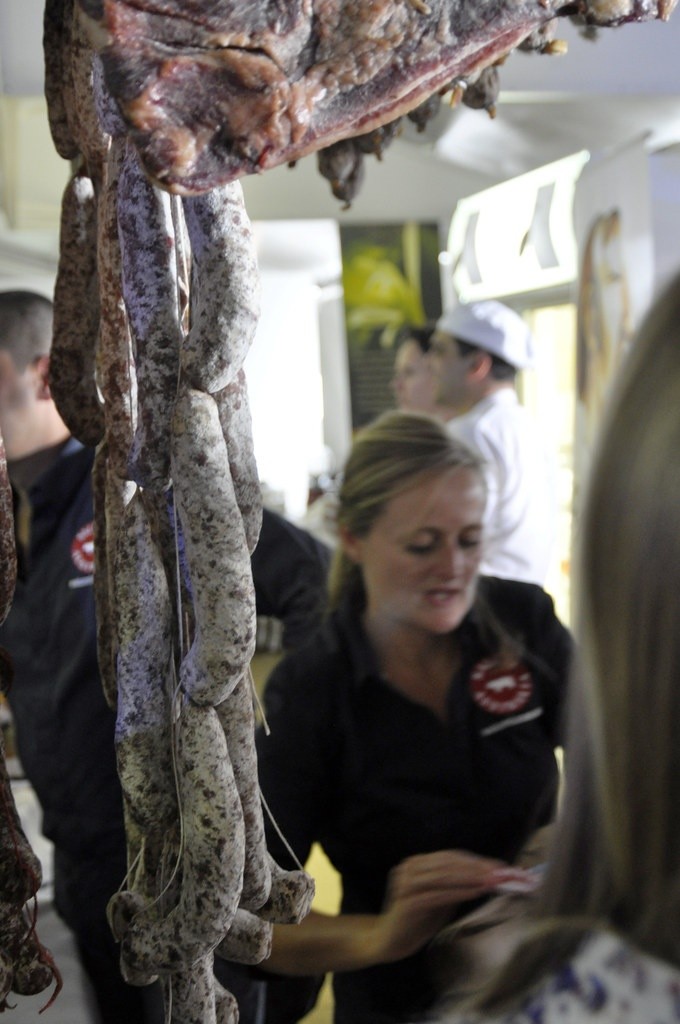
[
  {"x1": 0, "y1": 289, "x2": 335, "y2": 1024},
  {"x1": 223, "y1": 412, "x2": 574, "y2": 1024},
  {"x1": 443, "y1": 268, "x2": 680, "y2": 1024},
  {"x1": 388, "y1": 300, "x2": 564, "y2": 586}
]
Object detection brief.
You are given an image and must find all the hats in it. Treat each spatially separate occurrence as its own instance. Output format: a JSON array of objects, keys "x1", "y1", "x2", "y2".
[{"x1": 437, "y1": 299, "x2": 530, "y2": 375}]
